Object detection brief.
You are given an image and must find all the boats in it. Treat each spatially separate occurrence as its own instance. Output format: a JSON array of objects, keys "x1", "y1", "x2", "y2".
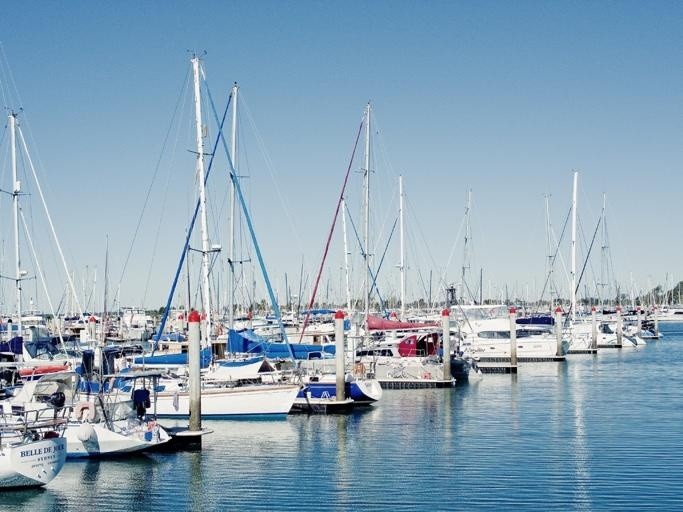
[
  {"x1": 0, "y1": 359, "x2": 172, "y2": 457},
  {"x1": 0, "y1": 402, "x2": 70, "y2": 492}
]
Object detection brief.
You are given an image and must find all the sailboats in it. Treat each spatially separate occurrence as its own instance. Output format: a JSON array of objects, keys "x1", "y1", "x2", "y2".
[{"x1": 0, "y1": 48, "x2": 683, "y2": 423}]
